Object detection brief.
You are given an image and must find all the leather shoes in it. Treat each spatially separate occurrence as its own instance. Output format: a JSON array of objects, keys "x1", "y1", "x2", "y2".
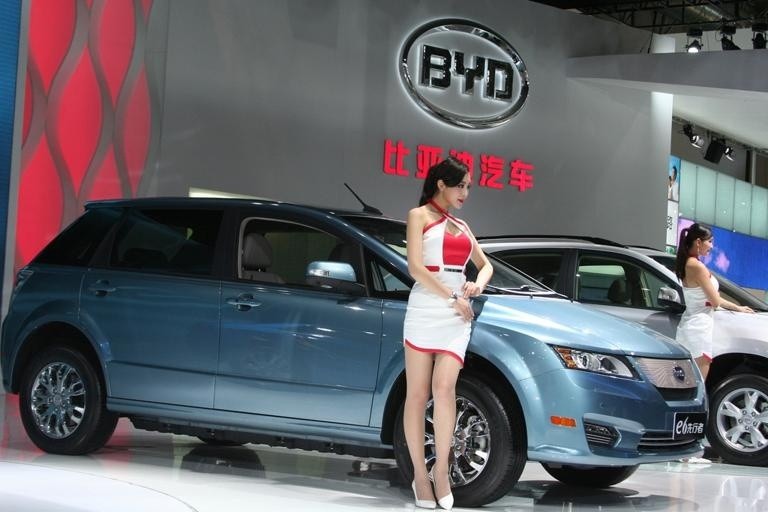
[
  {"x1": 428, "y1": 463, "x2": 454, "y2": 510},
  {"x1": 411, "y1": 480, "x2": 437, "y2": 509}
]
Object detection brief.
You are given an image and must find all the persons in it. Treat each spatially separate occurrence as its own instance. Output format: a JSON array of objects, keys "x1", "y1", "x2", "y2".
[
  {"x1": 670, "y1": 165, "x2": 679, "y2": 202},
  {"x1": 400, "y1": 154, "x2": 496, "y2": 512},
  {"x1": 673, "y1": 223, "x2": 759, "y2": 466},
  {"x1": 667, "y1": 176, "x2": 672, "y2": 200}
]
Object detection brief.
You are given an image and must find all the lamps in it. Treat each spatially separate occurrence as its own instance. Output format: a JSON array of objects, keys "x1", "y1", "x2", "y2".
[
  {"x1": 683, "y1": 23, "x2": 767, "y2": 54},
  {"x1": 681, "y1": 122, "x2": 735, "y2": 165}
]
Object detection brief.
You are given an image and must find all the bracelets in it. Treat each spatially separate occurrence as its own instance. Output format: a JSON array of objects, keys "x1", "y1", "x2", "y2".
[{"x1": 445, "y1": 290, "x2": 458, "y2": 307}]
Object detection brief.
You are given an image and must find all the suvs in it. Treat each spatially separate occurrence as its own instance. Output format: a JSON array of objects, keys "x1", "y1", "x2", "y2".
[{"x1": 1, "y1": 198, "x2": 709, "y2": 508}]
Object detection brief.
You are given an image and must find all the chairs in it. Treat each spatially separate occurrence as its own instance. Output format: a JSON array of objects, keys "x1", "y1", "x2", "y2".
[
  {"x1": 328, "y1": 244, "x2": 371, "y2": 290},
  {"x1": 606, "y1": 279, "x2": 632, "y2": 307},
  {"x1": 242, "y1": 231, "x2": 286, "y2": 284}
]
